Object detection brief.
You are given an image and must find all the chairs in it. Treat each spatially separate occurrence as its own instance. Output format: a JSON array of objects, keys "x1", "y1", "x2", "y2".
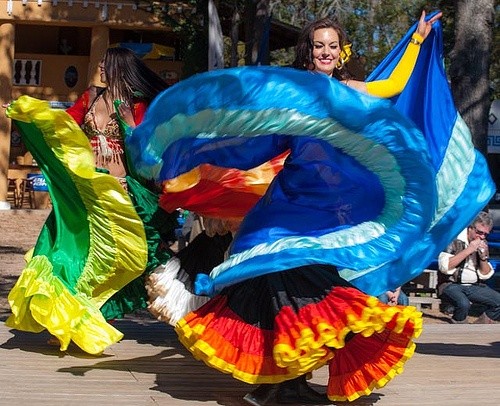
[
  {"x1": 5, "y1": 178, "x2": 19, "y2": 209},
  {"x1": 20, "y1": 179, "x2": 36, "y2": 208}
]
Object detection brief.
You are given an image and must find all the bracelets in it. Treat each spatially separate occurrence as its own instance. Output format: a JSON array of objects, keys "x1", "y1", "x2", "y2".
[
  {"x1": 410, "y1": 38, "x2": 419, "y2": 45},
  {"x1": 480, "y1": 258, "x2": 486, "y2": 262}
]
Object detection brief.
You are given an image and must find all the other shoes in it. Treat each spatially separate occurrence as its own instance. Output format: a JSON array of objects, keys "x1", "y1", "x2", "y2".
[{"x1": 242, "y1": 375, "x2": 335, "y2": 406}]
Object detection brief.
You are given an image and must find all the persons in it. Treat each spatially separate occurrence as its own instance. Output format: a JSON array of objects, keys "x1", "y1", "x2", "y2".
[
  {"x1": 439, "y1": 210, "x2": 500, "y2": 324},
  {"x1": 379, "y1": 286, "x2": 409, "y2": 306},
  {"x1": 128, "y1": 11, "x2": 497, "y2": 406},
  {"x1": 2, "y1": 46, "x2": 170, "y2": 357}
]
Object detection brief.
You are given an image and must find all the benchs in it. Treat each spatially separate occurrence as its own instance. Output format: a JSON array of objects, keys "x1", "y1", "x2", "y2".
[{"x1": 407, "y1": 269, "x2": 473, "y2": 309}]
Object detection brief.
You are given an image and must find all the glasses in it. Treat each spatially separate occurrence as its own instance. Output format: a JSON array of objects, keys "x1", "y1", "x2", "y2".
[{"x1": 471, "y1": 225, "x2": 490, "y2": 238}]
